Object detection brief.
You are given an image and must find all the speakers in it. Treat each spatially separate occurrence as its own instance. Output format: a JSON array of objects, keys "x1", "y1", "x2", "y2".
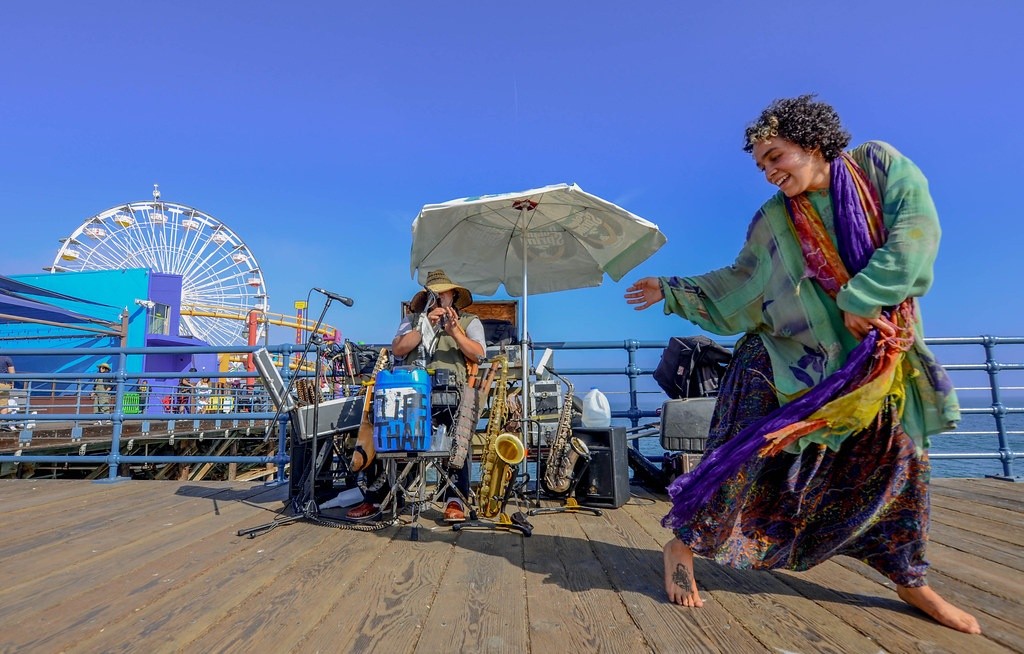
[
  {"x1": 572, "y1": 426, "x2": 630, "y2": 510},
  {"x1": 288, "y1": 416, "x2": 333, "y2": 506}
]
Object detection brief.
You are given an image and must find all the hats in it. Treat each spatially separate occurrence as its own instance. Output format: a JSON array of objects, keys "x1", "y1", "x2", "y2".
[
  {"x1": 409, "y1": 269, "x2": 473, "y2": 313},
  {"x1": 97, "y1": 363, "x2": 111, "y2": 371}
]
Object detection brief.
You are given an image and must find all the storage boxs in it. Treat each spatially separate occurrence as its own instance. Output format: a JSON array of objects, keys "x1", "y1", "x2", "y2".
[
  {"x1": 477, "y1": 345, "x2": 523, "y2": 369},
  {"x1": 529, "y1": 380, "x2": 563, "y2": 445},
  {"x1": 252, "y1": 347, "x2": 365, "y2": 441}
]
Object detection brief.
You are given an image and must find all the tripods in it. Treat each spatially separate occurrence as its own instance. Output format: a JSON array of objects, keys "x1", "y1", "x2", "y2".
[{"x1": 238, "y1": 302, "x2": 381, "y2": 537}]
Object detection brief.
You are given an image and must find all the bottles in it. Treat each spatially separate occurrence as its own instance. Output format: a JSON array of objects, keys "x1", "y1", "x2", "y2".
[
  {"x1": 318, "y1": 487, "x2": 365, "y2": 509},
  {"x1": 582, "y1": 386, "x2": 611, "y2": 428}
]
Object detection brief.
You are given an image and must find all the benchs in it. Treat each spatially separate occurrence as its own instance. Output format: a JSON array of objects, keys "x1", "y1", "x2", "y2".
[{"x1": 9, "y1": 391, "x2": 47, "y2": 413}]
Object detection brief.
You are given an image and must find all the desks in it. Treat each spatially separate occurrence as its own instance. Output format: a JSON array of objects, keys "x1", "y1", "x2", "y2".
[{"x1": 375, "y1": 451, "x2": 477, "y2": 540}]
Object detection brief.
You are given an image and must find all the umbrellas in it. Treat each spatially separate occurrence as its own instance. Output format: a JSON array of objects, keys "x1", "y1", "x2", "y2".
[{"x1": 410, "y1": 181, "x2": 668, "y2": 498}]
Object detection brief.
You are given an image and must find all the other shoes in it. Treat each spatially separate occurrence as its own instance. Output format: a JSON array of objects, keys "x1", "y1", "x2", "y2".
[
  {"x1": 94, "y1": 422, "x2": 112, "y2": 426},
  {"x1": 0, "y1": 424, "x2": 12, "y2": 432}
]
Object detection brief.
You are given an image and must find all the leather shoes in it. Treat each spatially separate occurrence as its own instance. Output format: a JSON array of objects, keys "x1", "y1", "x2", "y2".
[
  {"x1": 346, "y1": 503, "x2": 392, "y2": 519},
  {"x1": 444, "y1": 501, "x2": 466, "y2": 521}
]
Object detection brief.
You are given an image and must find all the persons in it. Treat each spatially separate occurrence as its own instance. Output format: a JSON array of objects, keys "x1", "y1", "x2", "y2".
[
  {"x1": 623, "y1": 90, "x2": 981, "y2": 636},
  {"x1": 174, "y1": 368, "x2": 212, "y2": 414},
  {"x1": 89, "y1": 363, "x2": 112, "y2": 425},
  {"x1": 346, "y1": 269, "x2": 487, "y2": 522},
  {"x1": 138, "y1": 379, "x2": 152, "y2": 414},
  {"x1": 0, "y1": 356, "x2": 16, "y2": 431}
]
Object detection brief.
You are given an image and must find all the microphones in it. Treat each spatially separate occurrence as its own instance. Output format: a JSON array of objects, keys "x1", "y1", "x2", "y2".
[
  {"x1": 425, "y1": 286, "x2": 439, "y2": 299},
  {"x1": 314, "y1": 288, "x2": 354, "y2": 307}
]
